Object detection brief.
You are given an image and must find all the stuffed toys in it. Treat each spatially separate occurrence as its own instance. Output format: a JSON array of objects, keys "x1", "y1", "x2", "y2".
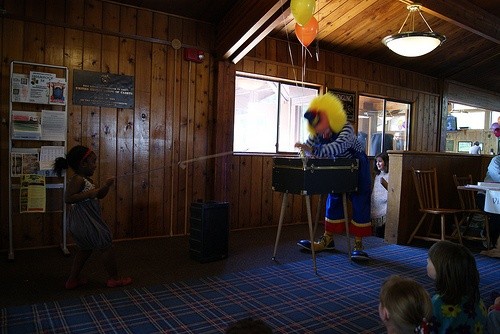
[{"x1": 292, "y1": 92, "x2": 372, "y2": 259}]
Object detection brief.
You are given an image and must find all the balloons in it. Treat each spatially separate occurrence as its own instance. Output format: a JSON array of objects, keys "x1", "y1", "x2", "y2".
[
  {"x1": 290, "y1": 0, "x2": 319, "y2": 48},
  {"x1": 490, "y1": 116, "x2": 500, "y2": 137}
]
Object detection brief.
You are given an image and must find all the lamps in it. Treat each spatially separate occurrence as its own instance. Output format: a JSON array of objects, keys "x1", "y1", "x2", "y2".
[{"x1": 380, "y1": 3, "x2": 447, "y2": 58}]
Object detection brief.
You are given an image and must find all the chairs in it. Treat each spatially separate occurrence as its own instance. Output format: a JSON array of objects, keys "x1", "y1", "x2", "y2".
[
  {"x1": 406, "y1": 167, "x2": 471, "y2": 247},
  {"x1": 448, "y1": 174, "x2": 493, "y2": 250}
]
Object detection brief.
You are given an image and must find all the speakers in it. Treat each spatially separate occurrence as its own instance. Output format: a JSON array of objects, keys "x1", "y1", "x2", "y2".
[{"x1": 190, "y1": 201, "x2": 229, "y2": 263}]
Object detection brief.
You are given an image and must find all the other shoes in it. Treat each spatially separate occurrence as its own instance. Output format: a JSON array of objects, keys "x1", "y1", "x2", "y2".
[
  {"x1": 480, "y1": 230, "x2": 493, "y2": 249},
  {"x1": 107, "y1": 276, "x2": 132, "y2": 287},
  {"x1": 64, "y1": 277, "x2": 88, "y2": 288},
  {"x1": 351, "y1": 240, "x2": 368, "y2": 259},
  {"x1": 297, "y1": 235, "x2": 335, "y2": 251}
]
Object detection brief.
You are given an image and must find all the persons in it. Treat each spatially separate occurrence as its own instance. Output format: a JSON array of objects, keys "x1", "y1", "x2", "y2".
[
  {"x1": 469, "y1": 141, "x2": 481, "y2": 155},
  {"x1": 379, "y1": 240, "x2": 500, "y2": 334},
  {"x1": 51, "y1": 146, "x2": 133, "y2": 289},
  {"x1": 370, "y1": 151, "x2": 388, "y2": 237},
  {"x1": 474, "y1": 155, "x2": 500, "y2": 247}
]
